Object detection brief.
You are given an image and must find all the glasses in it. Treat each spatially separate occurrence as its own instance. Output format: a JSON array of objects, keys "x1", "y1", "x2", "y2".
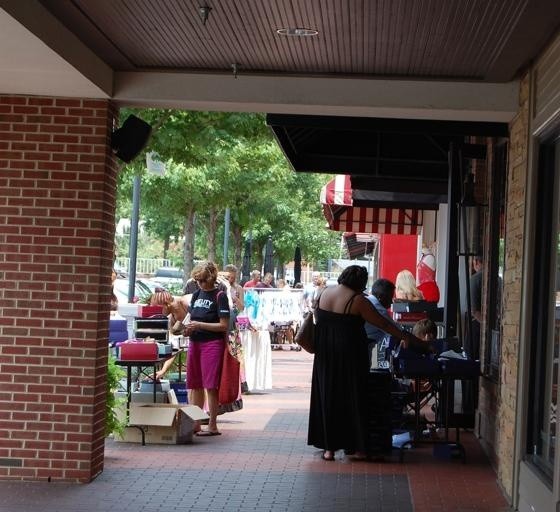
[{"x1": 194, "y1": 278, "x2": 207, "y2": 284}]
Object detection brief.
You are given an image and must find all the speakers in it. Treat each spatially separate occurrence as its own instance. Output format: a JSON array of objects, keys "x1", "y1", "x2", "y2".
[{"x1": 110, "y1": 114, "x2": 152, "y2": 164}]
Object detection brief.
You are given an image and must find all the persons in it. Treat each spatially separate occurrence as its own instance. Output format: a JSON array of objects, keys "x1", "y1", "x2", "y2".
[
  {"x1": 469, "y1": 252, "x2": 504, "y2": 355},
  {"x1": 244, "y1": 270, "x2": 326, "y2": 353},
  {"x1": 151, "y1": 262, "x2": 245, "y2": 435},
  {"x1": 308, "y1": 265, "x2": 440, "y2": 461}
]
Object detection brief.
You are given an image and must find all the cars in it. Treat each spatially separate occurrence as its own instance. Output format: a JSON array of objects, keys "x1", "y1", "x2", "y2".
[
  {"x1": 113, "y1": 264, "x2": 185, "y2": 306},
  {"x1": 270, "y1": 267, "x2": 296, "y2": 286}
]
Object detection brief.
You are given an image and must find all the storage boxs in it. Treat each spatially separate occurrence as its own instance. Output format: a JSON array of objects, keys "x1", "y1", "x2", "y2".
[
  {"x1": 118, "y1": 303, "x2": 172, "y2": 360},
  {"x1": 108, "y1": 380, "x2": 211, "y2": 450}
]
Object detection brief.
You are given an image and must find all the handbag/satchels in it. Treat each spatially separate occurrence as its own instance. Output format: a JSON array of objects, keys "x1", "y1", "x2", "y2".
[
  {"x1": 217, "y1": 345, "x2": 241, "y2": 405},
  {"x1": 294, "y1": 286, "x2": 327, "y2": 353}
]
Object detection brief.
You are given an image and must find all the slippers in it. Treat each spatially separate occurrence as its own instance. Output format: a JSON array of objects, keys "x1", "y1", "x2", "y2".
[
  {"x1": 197, "y1": 429, "x2": 222, "y2": 436},
  {"x1": 320, "y1": 450, "x2": 334, "y2": 461}
]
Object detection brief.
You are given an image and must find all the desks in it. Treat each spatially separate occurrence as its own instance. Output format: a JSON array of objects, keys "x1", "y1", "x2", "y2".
[{"x1": 113, "y1": 349, "x2": 183, "y2": 409}]
